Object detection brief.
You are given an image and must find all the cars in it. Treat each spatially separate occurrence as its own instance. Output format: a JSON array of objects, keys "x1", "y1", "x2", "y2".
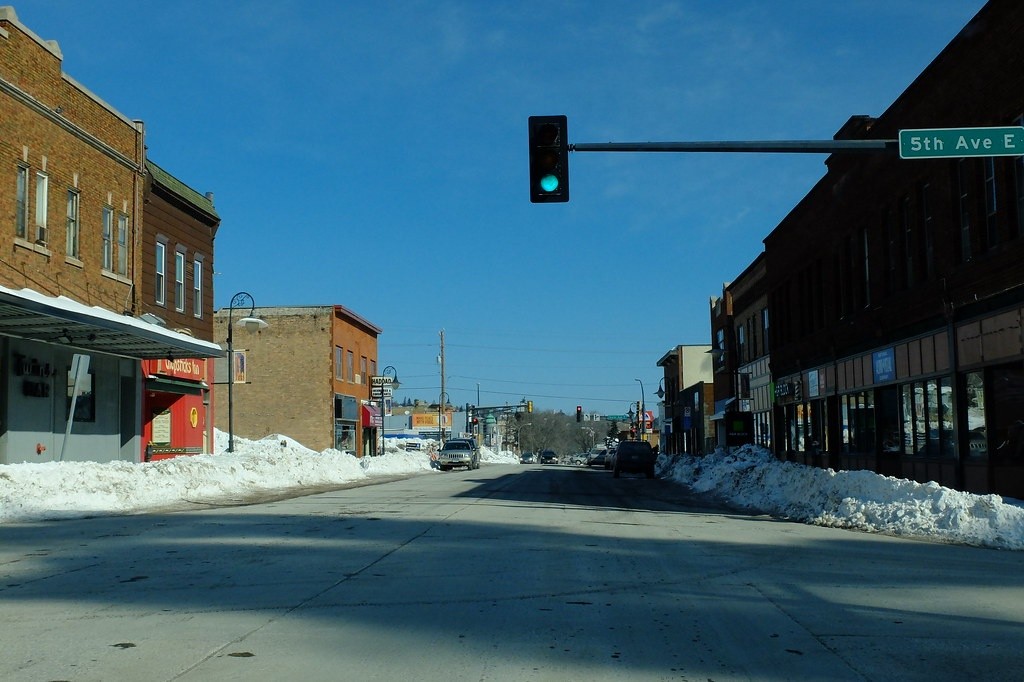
[
  {"x1": 569, "y1": 452, "x2": 589, "y2": 465},
  {"x1": 519, "y1": 451, "x2": 537, "y2": 464},
  {"x1": 603, "y1": 448, "x2": 617, "y2": 470},
  {"x1": 541, "y1": 451, "x2": 559, "y2": 464}
]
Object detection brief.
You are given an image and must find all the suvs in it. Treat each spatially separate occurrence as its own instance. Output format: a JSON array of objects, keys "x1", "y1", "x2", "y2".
[
  {"x1": 437, "y1": 440, "x2": 476, "y2": 470},
  {"x1": 450, "y1": 437, "x2": 482, "y2": 469},
  {"x1": 586, "y1": 448, "x2": 606, "y2": 466},
  {"x1": 609, "y1": 439, "x2": 656, "y2": 479}
]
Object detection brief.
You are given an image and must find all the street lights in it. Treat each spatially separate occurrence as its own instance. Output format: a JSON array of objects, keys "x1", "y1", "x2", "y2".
[
  {"x1": 651, "y1": 375, "x2": 676, "y2": 457},
  {"x1": 518, "y1": 423, "x2": 532, "y2": 461},
  {"x1": 380, "y1": 366, "x2": 403, "y2": 454},
  {"x1": 227, "y1": 292, "x2": 269, "y2": 453},
  {"x1": 436, "y1": 391, "x2": 452, "y2": 455},
  {"x1": 634, "y1": 378, "x2": 646, "y2": 441},
  {"x1": 627, "y1": 402, "x2": 641, "y2": 442}
]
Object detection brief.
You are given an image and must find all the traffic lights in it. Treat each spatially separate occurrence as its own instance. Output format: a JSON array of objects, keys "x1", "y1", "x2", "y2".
[
  {"x1": 472, "y1": 417, "x2": 478, "y2": 425},
  {"x1": 529, "y1": 115, "x2": 570, "y2": 202},
  {"x1": 577, "y1": 406, "x2": 582, "y2": 422},
  {"x1": 631, "y1": 430, "x2": 636, "y2": 438}
]
